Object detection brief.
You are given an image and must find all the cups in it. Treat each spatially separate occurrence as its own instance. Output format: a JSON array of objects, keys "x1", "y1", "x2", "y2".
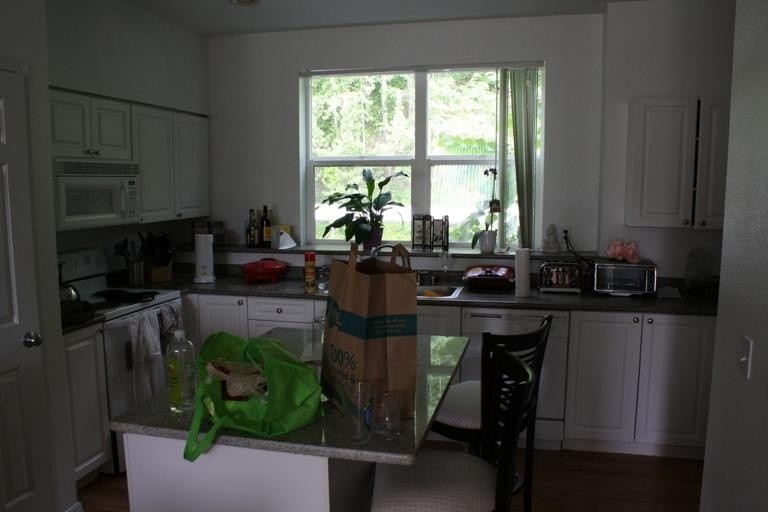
[
  {"x1": 364, "y1": 391, "x2": 403, "y2": 442},
  {"x1": 418, "y1": 270, "x2": 432, "y2": 286},
  {"x1": 340, "y1": 381, "x2": 370, "y2": 442},
  {"x1": 128, "y1": 260, "x2": 143, "y2": 287},
  {"x1": 315, "y1": 265, "x2": 330, "y2": 294}
]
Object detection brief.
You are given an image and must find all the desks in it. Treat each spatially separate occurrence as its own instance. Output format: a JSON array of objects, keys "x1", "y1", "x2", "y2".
[{"x1": 109, "y1": 325, "x2": 470, "y2": 512}]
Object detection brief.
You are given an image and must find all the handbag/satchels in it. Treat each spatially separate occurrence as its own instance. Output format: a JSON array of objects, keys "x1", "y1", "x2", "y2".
[
  {"x1": 183, "y1": 330, "x2": 326, "y2": 462},
  {"x1": 320, "y1": 241, "x2": 418, "y2": 441}
]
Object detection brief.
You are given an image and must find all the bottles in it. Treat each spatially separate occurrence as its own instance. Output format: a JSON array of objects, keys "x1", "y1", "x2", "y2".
[
  {"x1": 193, "y1": 219, "x2": 228, "y2": 246},
  {"x1": 303, "y1": 251, "x2": 316, "y2": 294},
  {"x1": 245, "y1": 208, "x2": 258, "y2": 248},
  {"x1": 260, "y1": 205, "x2": 271, "y2": 249},
  {"x1": 166, "y1": 329, "x2": 196, "y2": 414}
]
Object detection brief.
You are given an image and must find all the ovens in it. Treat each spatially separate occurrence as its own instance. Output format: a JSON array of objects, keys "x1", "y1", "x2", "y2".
[{"x1": 104, "y1": 297, "x2": 185, "y2": 416}]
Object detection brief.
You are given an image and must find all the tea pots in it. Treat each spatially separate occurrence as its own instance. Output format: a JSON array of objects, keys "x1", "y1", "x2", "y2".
[{"x1": 57, "y1": 277, "x2": 84, "y2": 319}]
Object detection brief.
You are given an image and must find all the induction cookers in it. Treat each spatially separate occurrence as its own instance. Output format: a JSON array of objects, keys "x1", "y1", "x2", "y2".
[{"x1": 89, "y1": 287, "x2": 157, "y2": 302}]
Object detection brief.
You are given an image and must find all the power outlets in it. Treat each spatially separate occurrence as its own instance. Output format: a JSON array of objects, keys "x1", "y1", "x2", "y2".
[{"x1": 559, "y1": 224, "x2": 572, "y2": 244}]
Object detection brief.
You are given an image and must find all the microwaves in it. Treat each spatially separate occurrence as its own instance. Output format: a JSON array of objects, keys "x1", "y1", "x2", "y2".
[
  {"x1": 592, "y1": 258, "x2": 658, "y2": 297},
  {"x1": 55, "y1": 157, "x2": 144, "y2": 230}
]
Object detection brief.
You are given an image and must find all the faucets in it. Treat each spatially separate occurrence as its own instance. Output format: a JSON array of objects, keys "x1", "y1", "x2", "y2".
[
  {"x1": 369, "y1": 244, "x2": 407, "y2": 266},
  {"x1": 416, "y1": 271, "x2": 429, "y2": 286}
]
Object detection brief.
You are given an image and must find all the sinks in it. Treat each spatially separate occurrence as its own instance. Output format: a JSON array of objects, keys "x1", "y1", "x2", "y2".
[{"x1": 416, "y1": 282, "x2": 465, "y2": 299}]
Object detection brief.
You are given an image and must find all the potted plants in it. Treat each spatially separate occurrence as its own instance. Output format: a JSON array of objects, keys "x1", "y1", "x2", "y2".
[
  {"x1": 469, "y1": 212, "x2": 499, "y2": 255},
  {"x1": 311, "y1": 166, "x2": 408, "y2": 253}
]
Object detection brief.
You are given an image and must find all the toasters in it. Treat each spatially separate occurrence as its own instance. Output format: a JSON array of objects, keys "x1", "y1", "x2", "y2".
[{"x1": 538, "y1": 260, "x2": 583, "y2": 294}]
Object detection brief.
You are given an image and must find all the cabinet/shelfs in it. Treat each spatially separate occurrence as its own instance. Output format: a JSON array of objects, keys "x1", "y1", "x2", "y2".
[
  {"x1": 62, "y1": 318, "x2": 113, "y2": 491},
  {"x1": 51, "y1": 84, "x2": 132, "y2": 160},
  {"x1": 559, "y1": 309, "x2": 716, "y2": 460},
  {"x1": 185, "y1": 294, "x2": 247, "y2": 354},
  {"x1": 131, "y1": 102, "x2": 212, "y2": 225},
  {"x1": 247, "y1": 293, "x2": 316, "y2": 357},
  {"x1": 625, "y1": 93, "x2": 732, "y2": 231}
]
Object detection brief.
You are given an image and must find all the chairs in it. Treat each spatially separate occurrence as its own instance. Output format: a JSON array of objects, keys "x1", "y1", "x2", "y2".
[
  {"x1": 423, "y1": 310, "x2": 554, "y2": 510},
  {"x1": 367, "y1": 342, "x2": 535, "y2": 512}
]
